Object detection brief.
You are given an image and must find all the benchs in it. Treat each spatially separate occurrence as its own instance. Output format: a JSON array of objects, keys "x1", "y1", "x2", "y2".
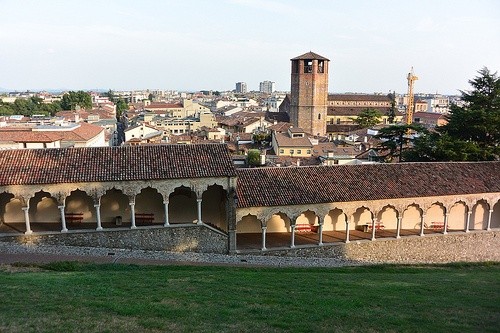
[
  {"x1": 60, "y1": 213, "x2": 84, "y2": 224},
  {"x1": 135, "y1": 214, "x2": 155, "y2": 226},
  {"x1": 366, "y1": 222, "x2": 385, "y2": 232},
  {"x1": 295, "y1": 224, "x2": 313, "y2": 233},
  {"x1": 431, "y1": 222, "x2": 448, "y2": 232}
]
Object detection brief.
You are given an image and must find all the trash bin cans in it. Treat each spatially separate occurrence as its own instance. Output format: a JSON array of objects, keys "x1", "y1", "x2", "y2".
[
  {"x1": 115, "y1": 216, "x2": 122, "y2": 226},
  {"x1": 363, "y1": 225, "x2": 369, "y2": 233}
]
